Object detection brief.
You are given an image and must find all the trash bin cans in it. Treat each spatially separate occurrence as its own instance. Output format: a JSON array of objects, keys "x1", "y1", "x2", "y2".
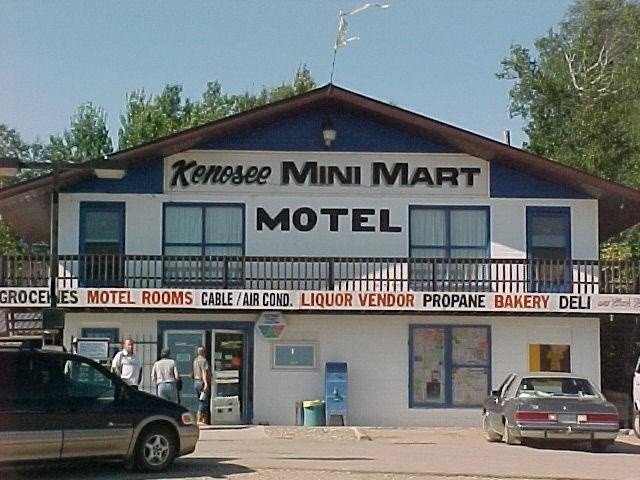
[{"x1": 303, "y1": 400, "x2": 325, "y2": 426}]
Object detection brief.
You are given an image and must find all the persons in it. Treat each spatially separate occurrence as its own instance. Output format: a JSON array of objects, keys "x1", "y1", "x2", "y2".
[
  {"x1": 111, "y1": 338, "x2": 143, "y2": 399},
  {"x1": 193, "y1": 347, "x2": 210, "y2": 426},
  {"x1": 151, "y1": 346, "x2": 181, "y2": 403}
]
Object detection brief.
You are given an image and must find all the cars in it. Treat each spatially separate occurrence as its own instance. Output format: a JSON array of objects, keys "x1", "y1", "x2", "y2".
[{"x1": 479, "y1": 369, "x2": 621, "y2": 455}]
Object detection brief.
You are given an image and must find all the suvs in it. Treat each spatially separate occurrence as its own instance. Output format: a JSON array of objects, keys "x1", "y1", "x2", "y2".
[{"x1": 0, "y1": 334, "x2": 205, "y2": 476}]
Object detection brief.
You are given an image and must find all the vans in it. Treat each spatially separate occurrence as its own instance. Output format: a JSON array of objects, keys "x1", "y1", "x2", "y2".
[{"x1": 631, "y1": 353, "x2": 640, "y2": 440}]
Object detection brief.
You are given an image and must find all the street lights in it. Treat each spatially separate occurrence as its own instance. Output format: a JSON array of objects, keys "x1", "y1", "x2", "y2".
[{"x1": 0, "y1": 155, "x2": 127, "y2": 304}]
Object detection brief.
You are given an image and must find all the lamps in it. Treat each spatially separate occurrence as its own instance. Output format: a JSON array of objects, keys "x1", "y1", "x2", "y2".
[{"x1": 322, "y1": 117, "x2": 337, "y2": 146}]
